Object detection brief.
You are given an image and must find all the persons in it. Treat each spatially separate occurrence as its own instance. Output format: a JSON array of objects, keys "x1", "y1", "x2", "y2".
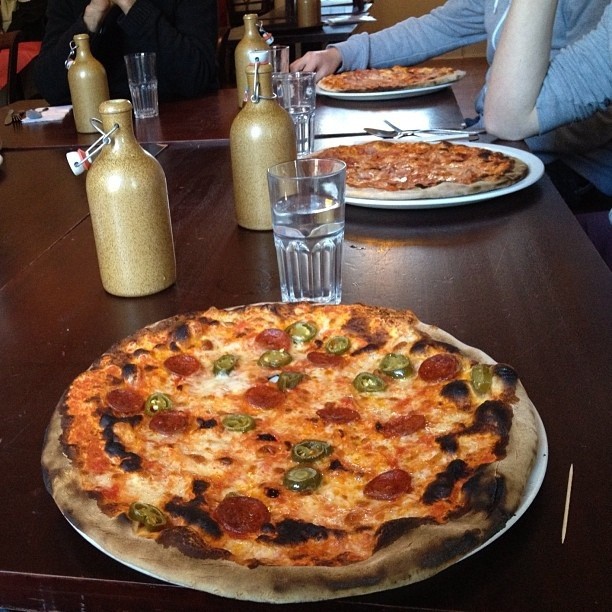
[
  {"x1": 289, "y1": 1, "x2": 611, "y2": 141},
  {"x1": 37, "y1": 1, "x2": 157, "y2": 101}
]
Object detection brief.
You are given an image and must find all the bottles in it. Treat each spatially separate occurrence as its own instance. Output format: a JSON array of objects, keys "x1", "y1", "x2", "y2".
[
  {"x1": 85, "y1": 99, "x2": 177, "y2": 299},
  {"x1": 67, "y1": 34, "x2": 110, "y2": 135},
  {"x1": 229, "y1": 50, "x2": 296, "y2": 233},
  {"x1": 235, "y1": 13, "x2": 270, "y2": 108}
]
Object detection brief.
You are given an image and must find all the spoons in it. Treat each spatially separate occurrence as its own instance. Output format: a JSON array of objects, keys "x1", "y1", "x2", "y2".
[{"x1": 364, "y1": 127, "x2": 487, "y2": 139}]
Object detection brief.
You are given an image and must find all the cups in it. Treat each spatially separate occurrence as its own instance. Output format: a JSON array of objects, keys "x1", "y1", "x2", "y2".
[
  {"x1": 124, "y1": 52, "x2": 164, "y2": 119},
  {"x1": 271, "y1": 45, "x2": 290, "y2": 74},
  {"x1": 273, "y1": 71, "x2": 317, "y2": 159},
  {"x1": 266, "y1": 159, "x2": 346, "y2": 307}
]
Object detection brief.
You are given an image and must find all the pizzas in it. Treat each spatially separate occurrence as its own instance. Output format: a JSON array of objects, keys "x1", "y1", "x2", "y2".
[
  {"x1": 317, "y1": 64, "x2": 468, "y2": 91},
  {"x1": 300, "y1": 140, "x2": 530, "y2": 199}
]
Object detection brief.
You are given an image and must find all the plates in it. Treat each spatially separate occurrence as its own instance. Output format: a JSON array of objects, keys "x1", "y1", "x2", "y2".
[
  {"x1": 322, "y1": 139, "x2": 545, "y2": 209},
  {"x1": 316, "y1": 71, "x2": 467, "y2": 101}
]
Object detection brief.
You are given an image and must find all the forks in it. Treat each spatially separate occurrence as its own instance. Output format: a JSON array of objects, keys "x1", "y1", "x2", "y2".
[{"x1": 384, "y1": 119, "x2": 480, "y2": 142}]
